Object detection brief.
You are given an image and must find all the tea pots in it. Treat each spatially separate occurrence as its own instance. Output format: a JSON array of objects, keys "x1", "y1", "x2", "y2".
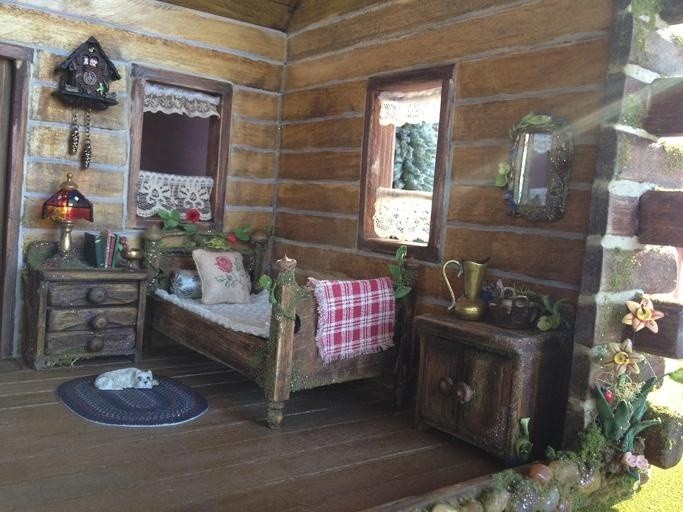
[{"x1": 442, "y1": 255, "x2": 492, "y2": 321}]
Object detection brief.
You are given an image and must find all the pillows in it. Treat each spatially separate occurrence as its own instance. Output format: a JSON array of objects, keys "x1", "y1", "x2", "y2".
[
  {"x1": 170, "y1": 267, "x2": 251, "y2": 299},
  {"x1": 193, "y1": 249, "x2": 252, "y2": 304}
]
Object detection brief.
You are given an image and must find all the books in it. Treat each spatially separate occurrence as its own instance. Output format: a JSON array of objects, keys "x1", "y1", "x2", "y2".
[{"x1": 84, "y1": 229, "x2": 120, "y2": 269}]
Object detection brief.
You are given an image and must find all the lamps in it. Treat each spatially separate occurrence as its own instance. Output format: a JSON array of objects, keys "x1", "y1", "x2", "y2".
[{"x1": 41, "y1": 173, "x2": 93, "y2": 270}]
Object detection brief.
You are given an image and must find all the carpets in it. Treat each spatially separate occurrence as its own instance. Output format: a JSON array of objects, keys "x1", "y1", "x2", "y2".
[{"x1": 56, "y1": 373, "x2": 208, "y2": 427}]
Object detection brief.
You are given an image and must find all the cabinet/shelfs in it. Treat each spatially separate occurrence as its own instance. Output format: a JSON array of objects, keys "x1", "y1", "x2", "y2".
[{"x1": 413, "y1": 309, "x2": 574, "y2": 469}]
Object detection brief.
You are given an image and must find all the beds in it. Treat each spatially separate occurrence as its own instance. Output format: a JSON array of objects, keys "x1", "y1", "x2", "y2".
[{"x1": 142, "y1": 222, "x2": 421, "y2": 431}]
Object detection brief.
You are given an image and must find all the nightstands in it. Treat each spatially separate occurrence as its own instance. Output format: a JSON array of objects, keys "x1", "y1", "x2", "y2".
[{"x1": 25, "y1": 241, "x2": 149, "y2": 370}]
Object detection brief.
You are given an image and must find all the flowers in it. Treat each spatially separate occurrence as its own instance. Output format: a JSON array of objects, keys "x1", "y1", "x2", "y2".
[
  {"x1": 593, "y1": 298, "x2": 662, "y2": 480},
  {"x1": 153, "y1": 207, "x2": 255, "y2": 249},
  {"x1": 213, "y1": 256, "x2": 251, "y2": 296}
]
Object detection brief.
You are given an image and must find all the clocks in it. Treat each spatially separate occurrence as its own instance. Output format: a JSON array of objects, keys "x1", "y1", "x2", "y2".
[{"x1": 52, "y1": 36, "x2": 121, "y2": 167}]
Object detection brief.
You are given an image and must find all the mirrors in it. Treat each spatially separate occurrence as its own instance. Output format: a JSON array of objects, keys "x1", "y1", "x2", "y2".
[{"x1": 494, "y1": 113, "x2": 573, "y2": 220}]
